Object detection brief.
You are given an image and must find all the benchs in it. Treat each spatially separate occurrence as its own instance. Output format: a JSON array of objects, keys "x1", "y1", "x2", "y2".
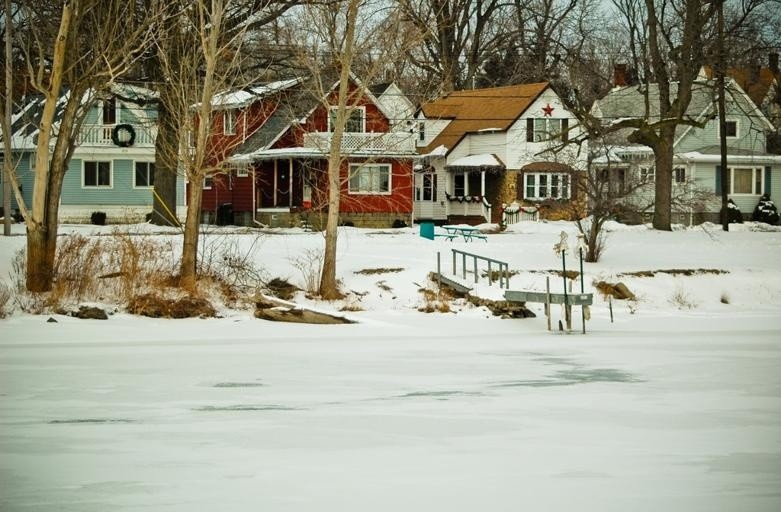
[
  {"x1": 433, "y1": 233, "x2": 459, "y2": 242},
  {"x1": 465, "y1": 233, "x2": 487, "y2": 243}
]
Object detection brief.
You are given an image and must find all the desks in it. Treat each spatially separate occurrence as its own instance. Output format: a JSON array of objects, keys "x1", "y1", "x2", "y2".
[{"x1": 442, "y1": 225, "x2": 479, "y2": 243}]
